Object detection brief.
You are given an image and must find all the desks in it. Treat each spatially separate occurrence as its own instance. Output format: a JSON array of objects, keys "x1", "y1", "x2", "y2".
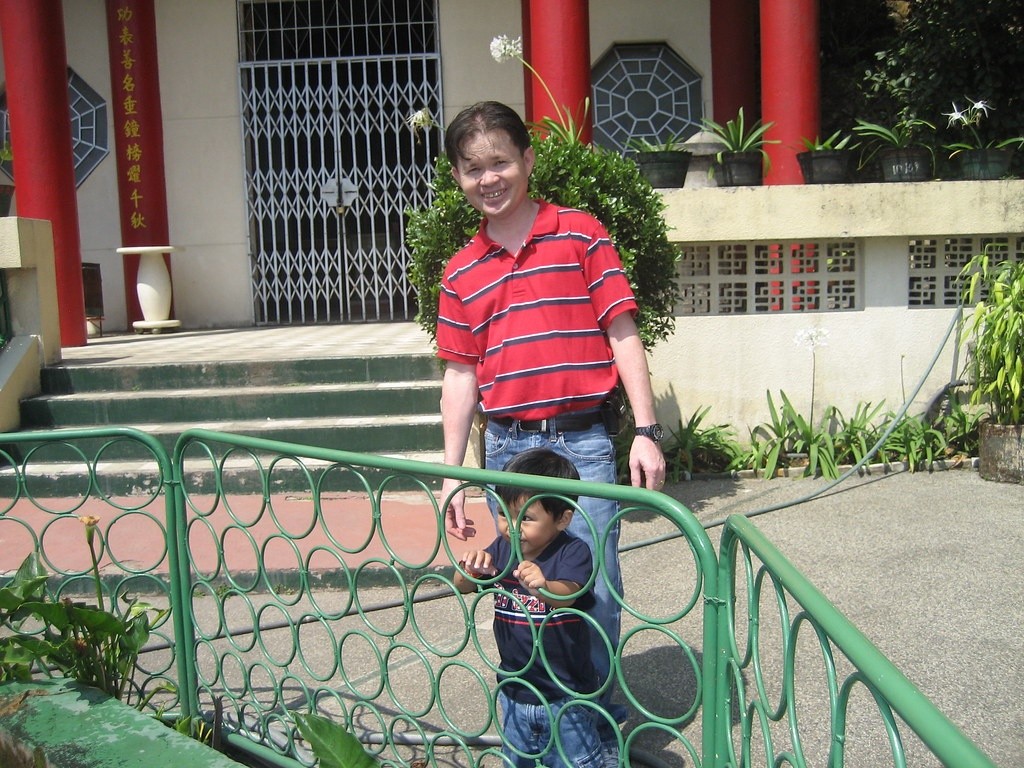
[{"x1": 115, "y1": 247, "x2": 181, "y2": 333}]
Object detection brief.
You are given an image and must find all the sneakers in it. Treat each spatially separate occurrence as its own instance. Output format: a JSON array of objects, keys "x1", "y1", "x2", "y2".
[{"x1": 601, "y1": 740, "x2": 619, "y2": 768}]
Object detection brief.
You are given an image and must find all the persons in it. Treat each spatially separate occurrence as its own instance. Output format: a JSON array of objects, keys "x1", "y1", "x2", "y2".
[
  {"x1": 453, "y1": 448, "x2": 606, "y2": 768},
  {"x1": 436, "y1": 101, "x2": 666, "y2": 768}
]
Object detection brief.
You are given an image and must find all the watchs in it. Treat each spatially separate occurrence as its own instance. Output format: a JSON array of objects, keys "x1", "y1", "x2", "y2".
[{"x1": 635, "y1": 424, "x2": 664, "y2": 444}]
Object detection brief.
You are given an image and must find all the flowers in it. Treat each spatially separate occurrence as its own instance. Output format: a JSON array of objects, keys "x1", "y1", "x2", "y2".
[{"x1": 940, "y1": 95, "x2": 1024, "y2": 159}]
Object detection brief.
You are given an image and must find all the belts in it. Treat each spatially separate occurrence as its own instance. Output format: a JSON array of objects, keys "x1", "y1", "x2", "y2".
[{"x1": 486, "y1": 410, "x2": 606, "y2": 433}]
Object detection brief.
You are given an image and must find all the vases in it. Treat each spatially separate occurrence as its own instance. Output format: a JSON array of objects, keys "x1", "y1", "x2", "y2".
[{"x1": 954, "y1": 148, "x2": 1015, "y2": 180}]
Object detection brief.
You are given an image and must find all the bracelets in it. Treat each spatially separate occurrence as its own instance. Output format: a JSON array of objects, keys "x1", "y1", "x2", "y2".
[{"x1": 459, "y1": 560, "x2": 483, "y2": 579}]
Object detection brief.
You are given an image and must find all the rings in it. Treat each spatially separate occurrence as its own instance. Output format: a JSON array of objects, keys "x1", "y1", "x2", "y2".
[{"x1": 657, "y1": 480, "x2": 664, "y2": 488}]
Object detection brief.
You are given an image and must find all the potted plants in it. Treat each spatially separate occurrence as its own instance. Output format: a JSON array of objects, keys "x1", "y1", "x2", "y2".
[
  {"x1": 0, "y1": 141, "x2": 16, "y2": 217},
  {"x1": 700, "y1": 106, "x2": 782, "y2": 187},
  {"x1": 620, "y1": 133, "x2": 693, "y2": 188},
  {"x1": 787, "y1": 129, "x2": 863, "y2": 185},
  {"x1": 955, "y1": 244, "x2": 1024, "y2": 482},
  {"x1": 851, "y1": 118, "x2": 937, "y2": 182}
]
